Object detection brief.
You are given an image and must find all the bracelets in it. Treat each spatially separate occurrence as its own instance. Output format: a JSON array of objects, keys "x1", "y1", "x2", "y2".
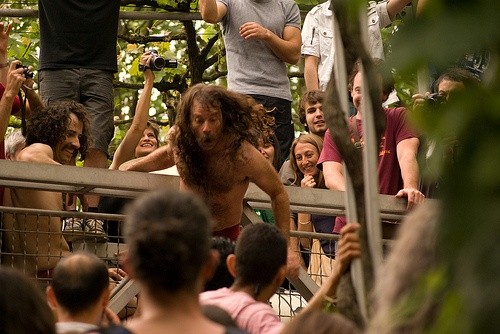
[
  {"x1": 297, "y1": 220, "x2": 312, "y2": 226},
  {"x1": 1, "y1": 62, "x2": 10, "y2": 69}
]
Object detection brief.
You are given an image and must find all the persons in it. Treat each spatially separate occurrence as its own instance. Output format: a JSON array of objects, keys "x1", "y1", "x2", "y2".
[
  {"x1": 1, "y1": 186, "x2": 276, "y2": 334},
  {"x1": 2, "y1": 100, "x2": 129, "y2": 290},
  {"x1": 200, "y1": 0, "x2": 302, "y2": 171},
  {"x1": 250, "y1": 129, "x2": 281, "y2": 223},
  {"x1": 298, "y1": 0, "x2": 412, "y2": 111},
  {"x1": 277, "y1": 56, "x2": 500, "y2": 334},
  {"x1": 121, "y1": 83, "x2": 301, "y2": 280},
  {"x1": 98, "y1": 51, "x2": 179, "y2": 244},
  {"x1": 4, "y1": 128, "x2": 28, "y2": 162},
  {"x1": 35, "y1": 0, "x2": 119, "y2": 238},
  {"x1": 0, "y1": 22, "x2": 15, "y2": 86},
  {"x1": 0, "y1": 61, "x2": 46, "y2": 143}
]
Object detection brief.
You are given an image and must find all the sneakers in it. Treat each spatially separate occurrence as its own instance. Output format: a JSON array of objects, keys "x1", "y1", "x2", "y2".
[
  {"x1": 63, "y1": 218, "x2": 83, "y2": 242},
  {"x1": 85, "y1": 218, "x2": 109, "y2": 242}
]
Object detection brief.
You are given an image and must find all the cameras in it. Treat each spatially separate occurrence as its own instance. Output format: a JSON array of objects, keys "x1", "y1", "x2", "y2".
[
  {"x1": 147, "y1": 49, "x2": 177, "y2": 71},
  {"x1": 15, "y1": 64, "x2": 34, "y2": 78},
  {"x1": 426, "y1": 92, "x2": 442, "y2": 109}
]
